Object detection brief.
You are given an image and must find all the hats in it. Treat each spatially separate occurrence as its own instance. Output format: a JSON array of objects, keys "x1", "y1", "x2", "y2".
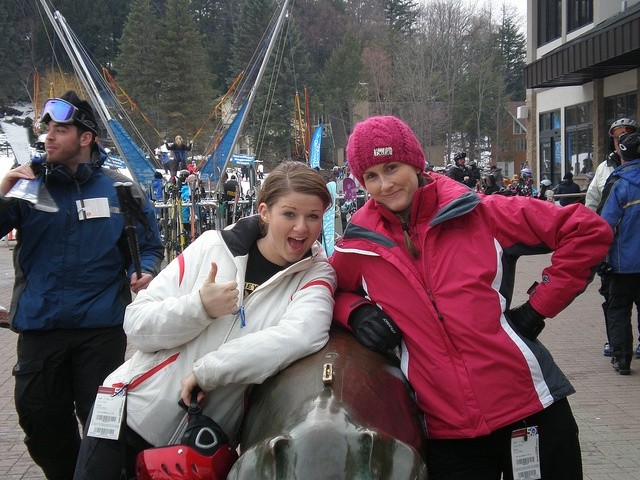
[
  {"x1": 44, "y1": 91, "x2": 99, "y2": 137},
  {"x1": 154, "y1": 172, "x2": 162, "y2": 179},
  {"x1": 453, "y1": 152, "x2": 466, "y2": 161},
  {"x1": 610, "y1": 118, "x2": 637, "y2": 131},
  {"x1": 346, "y1": 116, "x2": 425, "y2": 189},
  {"x1": 540, "y1": 179, "x2": 552, "y2": 186},
  {"x1": 563, "y1": 172, "x2": 572, "y2": 179},
  {"x1": 618, "y1": 132, "x2": 640, "y2": 160}
]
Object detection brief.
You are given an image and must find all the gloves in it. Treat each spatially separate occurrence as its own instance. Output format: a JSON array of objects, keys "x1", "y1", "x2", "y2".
[
  {"x1": 510, "y1": 300, "x2": 547, "y2": 341},
  {"x1": 596, "y1": 262, "x2": 612, "y2": 278},
  {"x1": 349, "y1": 303, "x2": 401, "y2": 351}
]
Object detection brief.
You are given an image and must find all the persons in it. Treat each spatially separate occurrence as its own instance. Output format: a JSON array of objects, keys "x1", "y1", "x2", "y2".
[
  {"x1": 216, "y1": 172, "x2": 228, "y2": 194},
  {"x1": 0, "y1": 91, "x2": 164, "y2": 480},
  {"x1": 177, "y1": 177, "x2": 191, "y2": 223},
  {"x1": 224, "y1": 175, "x2": 238, "y2": 200},
  {"x1": 447, "y1": 153, "x2": 475, "y2": 187},
  {"x1": 585, "y1": 118, "x2": 640, "y2": 374},
  {"x1": 554, "y1": 172, "x2": 580, "y2": 206},
  {"x1": 156, "y1": 151, "x2": 171, "y2": 174},
  {"x1": 150, "y1": 172, "x2": 164, "y2": 214},
  {"x1": 330, "y1": 115, "x2": 613, "y2": 478},
  {"x1": 163, "y1": 136, "x2": 193, "y2": 176},
  {"x1": 257, "y1": 163, "x2": 263, "y2": 177},
  {"x1": 72, "y1": 161, "x2": 337, "y2": 480},
  {"x1": 491, "y1": 165, "x2": 501, "y2": 185}
]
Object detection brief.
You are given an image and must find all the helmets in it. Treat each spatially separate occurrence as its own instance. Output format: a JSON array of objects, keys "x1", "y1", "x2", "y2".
[
  {"x1": 521, "y1": 168, "x2": 531, "y2": 177},
  {"x1": 135, "y1": 386, "x2": 240, "y2": 480}
]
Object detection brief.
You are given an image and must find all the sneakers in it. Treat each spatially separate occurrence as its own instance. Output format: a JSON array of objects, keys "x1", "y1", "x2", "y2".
[
  {"x1": 604, "y1": 343, "x2": 611, "y2": 356},
  {"x1": 611, "y1": 356, "x2": 632, "y2": 376},
  {"x1": 636, "y1": 345, "x2": 640, "y2": 358}
]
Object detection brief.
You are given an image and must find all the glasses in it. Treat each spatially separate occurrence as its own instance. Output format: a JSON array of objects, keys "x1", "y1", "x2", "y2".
[{"x1": 39, "y1": 98, "x2": 98, "y2": 135}]
